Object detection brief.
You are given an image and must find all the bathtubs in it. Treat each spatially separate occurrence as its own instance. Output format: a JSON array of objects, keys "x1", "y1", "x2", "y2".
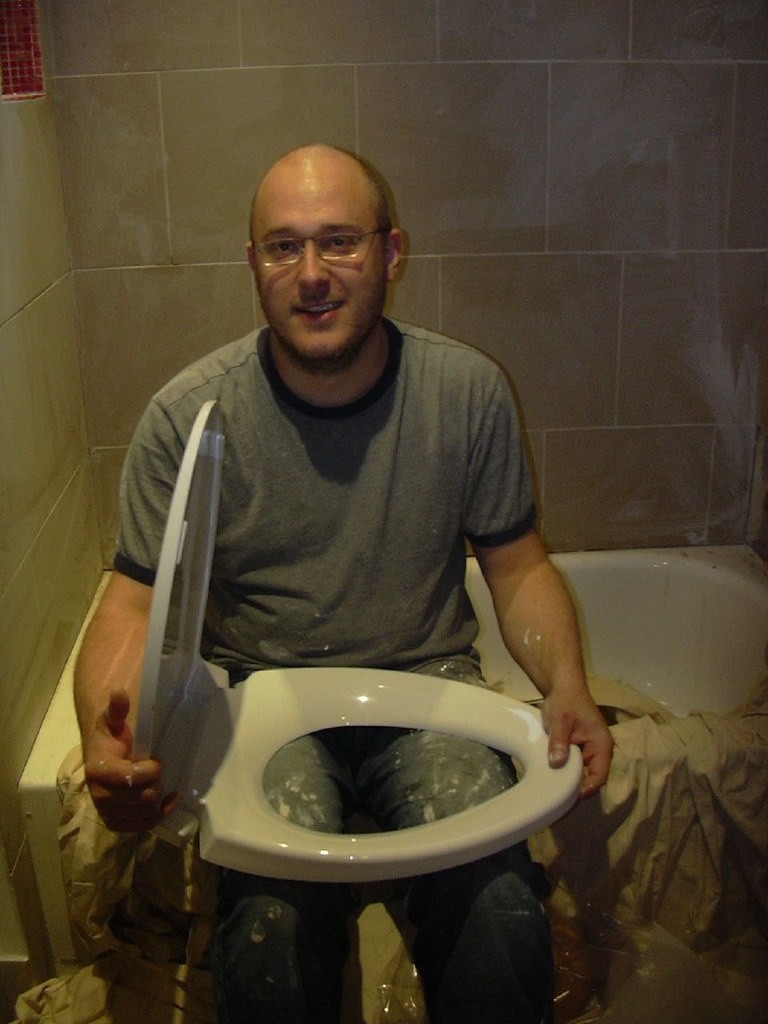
[{"x1": 14, "y1": 542, "x2": 768, "y2": 972}]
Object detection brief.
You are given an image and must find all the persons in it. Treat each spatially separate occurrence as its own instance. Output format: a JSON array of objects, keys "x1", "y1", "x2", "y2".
[{"x1": 74, "y1": 144, "x2": 614, "y2": 1024}]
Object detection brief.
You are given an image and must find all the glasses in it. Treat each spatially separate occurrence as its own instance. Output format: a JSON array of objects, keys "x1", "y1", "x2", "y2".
[{"x1": 255, "y1": 229, "x2": 389, "y2": 265}]
